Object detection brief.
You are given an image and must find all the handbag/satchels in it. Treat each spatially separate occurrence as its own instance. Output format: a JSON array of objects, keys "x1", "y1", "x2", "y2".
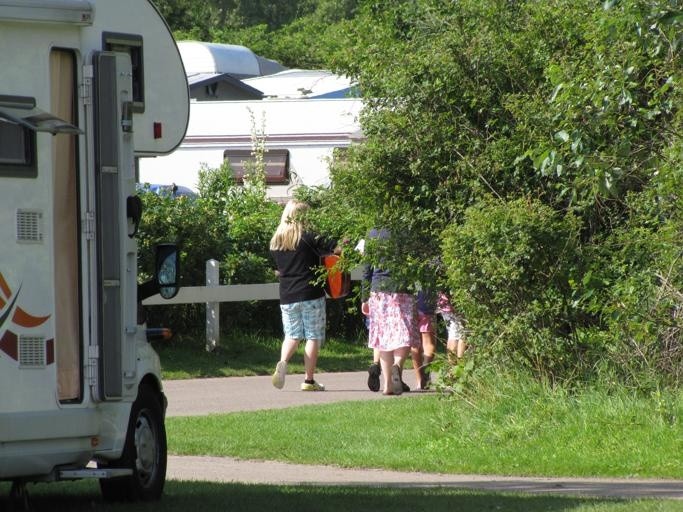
[{"x1": 319, "y1": 254, "x2": 351, "y2": 298}]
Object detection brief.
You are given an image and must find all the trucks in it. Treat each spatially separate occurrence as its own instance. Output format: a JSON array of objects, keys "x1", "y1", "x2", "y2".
[{"x1": 0, "y1": 1, "x2": 190, "y2": 502}]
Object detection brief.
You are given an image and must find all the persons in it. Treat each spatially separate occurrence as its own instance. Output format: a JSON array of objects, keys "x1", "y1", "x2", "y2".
[
  {"x1": 361, "y1": 229, "x2": 468, "y2": 395},
  {"x1": 268, "y1": 198, "x2": 342, "y2": 391}
]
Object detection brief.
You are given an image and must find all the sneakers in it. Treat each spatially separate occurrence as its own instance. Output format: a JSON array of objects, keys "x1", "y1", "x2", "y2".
[
  {"x1": 367, "y1": 362, "x2": 410, "y2": 395},
  {"x1": 418, "y1": 364, "x2": 431, "y2": 390},
  {"x1": 301, "y1": 381, "x2": 325, "y2": 392},
  {"x1": 272, "y1": 360, "x2": 287, "y2": 389}
]
